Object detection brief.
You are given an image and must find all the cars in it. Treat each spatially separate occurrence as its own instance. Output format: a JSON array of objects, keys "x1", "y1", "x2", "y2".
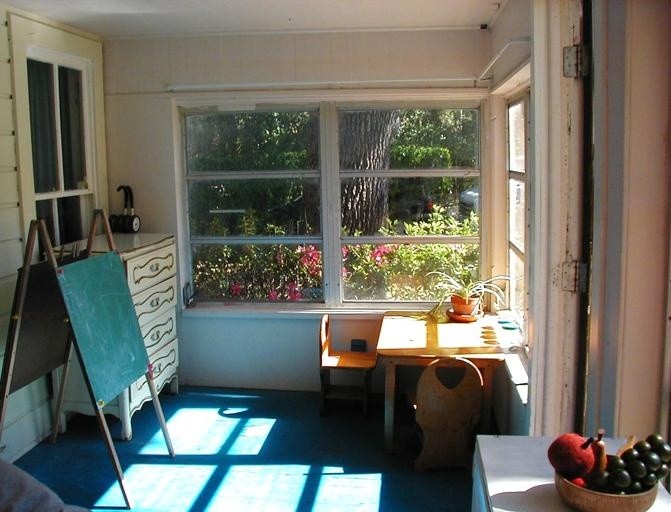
[{"x1": 458, "y1": 180, "x2": 520, "y2": 218}]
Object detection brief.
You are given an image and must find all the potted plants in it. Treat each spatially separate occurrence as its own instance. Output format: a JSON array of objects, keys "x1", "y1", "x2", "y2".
[{"x1": 422, "y1": 271, "x2": 511, "y2": 315}]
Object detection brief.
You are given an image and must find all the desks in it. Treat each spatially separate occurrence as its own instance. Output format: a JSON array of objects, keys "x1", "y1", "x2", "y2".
[{"x1": 375, "y1": 311, "x2": 503, "y2": 453}]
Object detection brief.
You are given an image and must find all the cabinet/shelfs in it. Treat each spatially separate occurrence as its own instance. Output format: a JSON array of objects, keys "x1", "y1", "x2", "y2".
[
  {"x1": 42, "y1": 229, "x2": 178, "y2": 444},
  {"x1": 471, "y1": 433, "x2": 670, "y2": 511}
]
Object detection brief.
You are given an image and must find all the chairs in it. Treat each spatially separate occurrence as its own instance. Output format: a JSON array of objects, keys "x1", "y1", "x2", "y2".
[
  {"x1": 406, "y1": 356, "x2": 484, "y2": 475},
  {"x1": 318, "y1": 314, "x2": 378, "y2": 418}
]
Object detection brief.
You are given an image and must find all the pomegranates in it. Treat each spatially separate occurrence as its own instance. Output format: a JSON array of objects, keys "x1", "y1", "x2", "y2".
[
  {"x1": 568, "y1": 476, "x2": 586, "y2": 488},
  {"x1": 547, "y1": 433, "x2": 596, "y2": 478}
]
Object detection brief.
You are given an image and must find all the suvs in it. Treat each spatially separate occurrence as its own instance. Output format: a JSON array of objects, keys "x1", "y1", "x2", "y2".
[{"x1": 400, "y1": 183, "x2": 453, "y2": 221}]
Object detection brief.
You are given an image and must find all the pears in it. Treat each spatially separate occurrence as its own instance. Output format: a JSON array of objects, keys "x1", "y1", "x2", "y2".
[{"x1": 589, "y1": 429, "x2": 608, "y2": 475}]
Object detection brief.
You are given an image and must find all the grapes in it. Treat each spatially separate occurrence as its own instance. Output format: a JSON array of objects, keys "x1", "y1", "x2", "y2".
[{"x1": 604, "y1": 432, "x2": 671, "y2": 495}]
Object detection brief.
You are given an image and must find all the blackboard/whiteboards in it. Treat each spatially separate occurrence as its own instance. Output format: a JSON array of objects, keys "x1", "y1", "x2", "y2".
[
  {"x1": 1, "y1": 247, "x2": 91, "y2": 400},
  {"x1": 55, "y1": 249, "x2": 151, "y2": 410}
]
